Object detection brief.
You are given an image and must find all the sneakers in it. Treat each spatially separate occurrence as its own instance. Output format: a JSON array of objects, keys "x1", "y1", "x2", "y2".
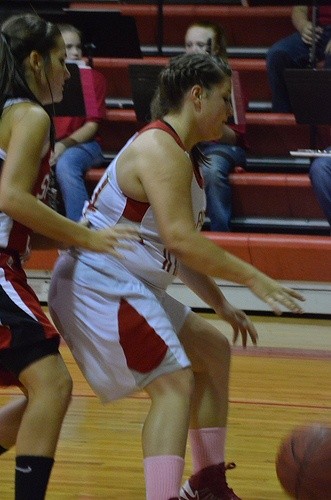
[{"x1": 180, "y1": 461, "x2": 242, "y2": 500}]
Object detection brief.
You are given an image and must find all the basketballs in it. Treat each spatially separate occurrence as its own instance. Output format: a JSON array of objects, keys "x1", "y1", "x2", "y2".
[{"x1": 275, "y1": 422, "x2": 331, "y2": 500}]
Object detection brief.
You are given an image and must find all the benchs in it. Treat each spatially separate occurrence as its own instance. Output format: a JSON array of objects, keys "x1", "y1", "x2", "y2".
[{"x1": 0, "y1": 0, "x2": 331, "y2": 317}]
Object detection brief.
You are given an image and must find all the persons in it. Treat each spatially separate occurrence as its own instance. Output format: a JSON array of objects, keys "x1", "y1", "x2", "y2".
[
  {"x1": 181, "y1": 24, "x2": 249, "y2": 234},
  {"x1": 308, "y1": 146, "x2": 331, "y2": 226},
  {"x1": 48, "y1": 49, "x2": 305, "y2": 500},
  {"x1": 264, "y1": 6, "x2": 331, "y2": 113},
  {"x1": 41, "y1": 23, "x2": 105, "y2": 223},
  {"x1": 0, "y1": 14, "x2": 141, "y2": 500}
]
